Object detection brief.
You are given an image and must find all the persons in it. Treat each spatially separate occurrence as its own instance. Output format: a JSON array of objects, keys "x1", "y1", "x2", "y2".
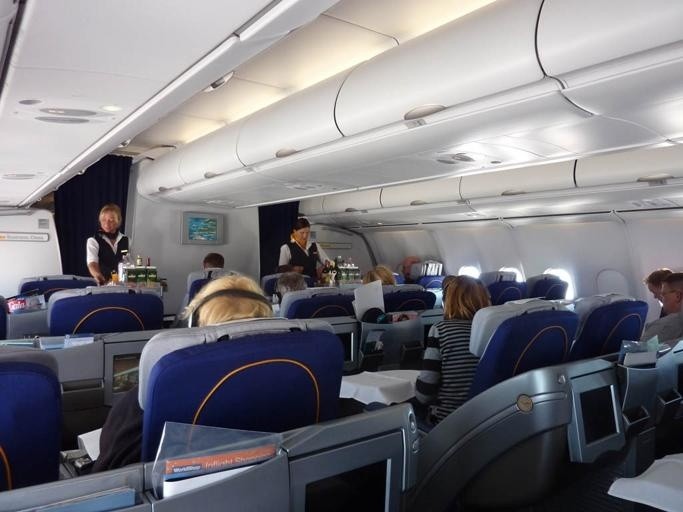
[
  {"x1": 279, "y1": 217, "x2": 334, "y2": 288},
  {"x1": 414, "y1": 275, "x2": 492, "y2": 427},
  {"x1": 203, "y1": 252, "x2": 224, "y2": 270},
  {"x1": 400, "y1": 257, "x2": 421, "y2": 284},
  {"x1": 183, "y1": 273, "x2": 274, "y2": 328},
  {"x1": 274, "y1": 265, "x2": 295, "y2": 274},
  {"x1": 441, "y1": 275, "x2": 457, "y2": 290},
  {"x1": 643, "y1": 268, "x2": 674, "y2": 319},
  {"x1": 639, "y1": 273, "x2": 683, "y2": 342},
  {"x1": 272, "y1": 273, "x2": 308, "y2": 309},
  {"x1": 86, "y1": 203, "x2": 134, "y2": 287},
  {"x1": 362, "y1": 264, "x2": 396, "y2": 286}
]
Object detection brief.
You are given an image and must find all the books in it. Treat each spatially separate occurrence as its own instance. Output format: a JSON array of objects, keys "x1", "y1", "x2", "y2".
[
  {"x1": 388, "y1": 310, "x2": 417, "y2": 324},
  {"x1": 4, "y1": 288, "x2": 48, "y2": 315},
  {"x1": 657, "y1": 343, "x2": 672, "y2": 356},
  {"x1": 39, "y1": 336, "x2": 95, "y2": 352},
  {"x1": 0, "y1": 338, "x2": 36, "y2": 354},
  {"x1": 410, "y1": 259, "x2": 444, "y2": 281},
  {"x1": 9, "y1": 485, "x2": 136, "y2": 512},
  {"x1": 162, "y1": 442, "x2": 278, "y2": 500},
  {"x1": 622, "y1": 351, "x2": 657, "y2": 366}
]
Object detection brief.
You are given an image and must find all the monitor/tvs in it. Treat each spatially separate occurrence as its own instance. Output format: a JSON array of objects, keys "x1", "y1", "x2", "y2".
[
  {"x1": 335, "y1": 332, "x2": 356, "y2": 361},
  {"x1": 569, "y1": 371, "x2": 628, "y2": 463},
  {"x1": 105, "y1": 341, "x2": 147, "y2": 403},
  {"x1": 181, "y1": 209, "x2": 224, "y2": 245},
  {"x1": 289, "y1": 431, "x2": 402, "y2": 511}
]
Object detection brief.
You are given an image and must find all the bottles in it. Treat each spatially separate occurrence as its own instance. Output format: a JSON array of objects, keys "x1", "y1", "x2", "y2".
[{"x1": 136, "y1": 254, "x2": 142, "y2": 266}]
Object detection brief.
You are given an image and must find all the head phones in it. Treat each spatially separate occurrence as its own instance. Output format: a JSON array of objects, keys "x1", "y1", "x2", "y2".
[
  {"x1": 439, "y1": 276, "x2": 468, "y2": 309},
  {"x1": 184, "y1": 288, "x2": 275, "y2": 329}
]
